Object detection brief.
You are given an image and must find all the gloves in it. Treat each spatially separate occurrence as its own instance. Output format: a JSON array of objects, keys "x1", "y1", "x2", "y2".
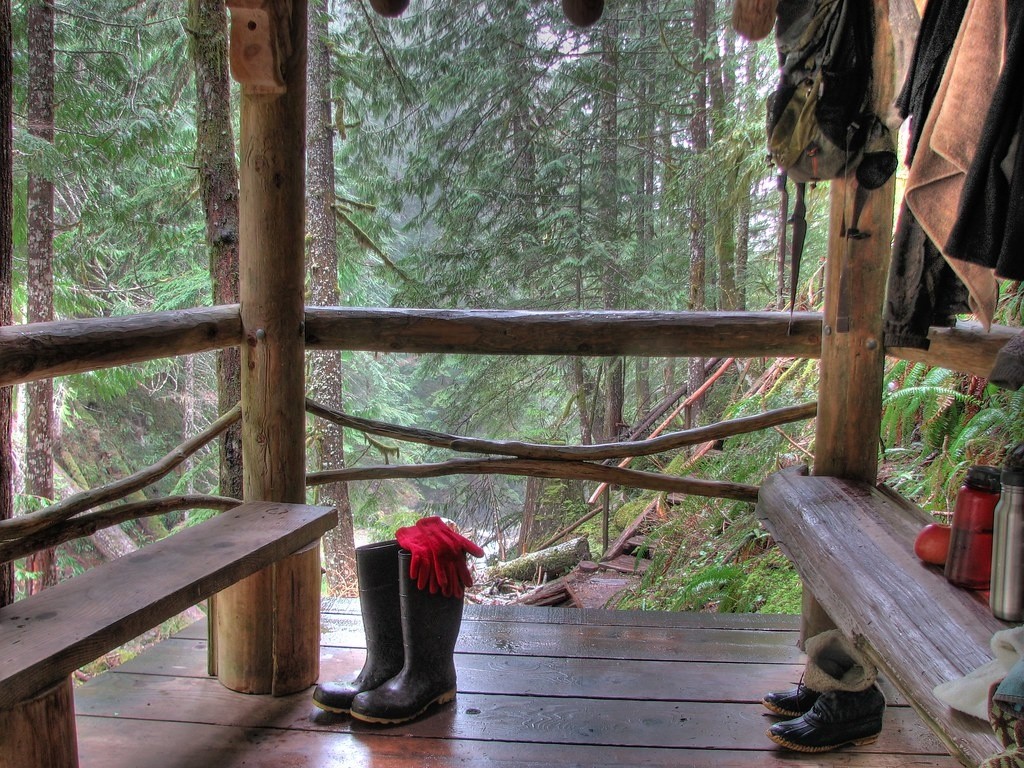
[{"x1": 395, "y1": 515, "x2": 485, "y2": 599}]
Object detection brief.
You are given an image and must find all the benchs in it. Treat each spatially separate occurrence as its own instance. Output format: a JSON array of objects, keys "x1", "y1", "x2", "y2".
[
  {"x1": 1, "y1": 496, "x2": 345, "y2": 768},
  {"x1": 753, "y1": 472, "x2": 1024, "y2": 768}
]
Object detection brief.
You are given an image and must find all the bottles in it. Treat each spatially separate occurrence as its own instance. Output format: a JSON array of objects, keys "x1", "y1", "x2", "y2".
[{"x1": 943, "y1": 465, "x2": 1000, "y2": 591}]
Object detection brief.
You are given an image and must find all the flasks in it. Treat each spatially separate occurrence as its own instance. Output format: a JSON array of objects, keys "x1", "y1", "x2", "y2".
[{"x1": 989, "y1": 454, "x2": 1024, "y2": 623}]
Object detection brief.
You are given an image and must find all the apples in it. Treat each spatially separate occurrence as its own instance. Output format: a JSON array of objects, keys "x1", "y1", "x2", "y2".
[{"x1": 915, "y1": 522, "x2": 952, "y2": 565}]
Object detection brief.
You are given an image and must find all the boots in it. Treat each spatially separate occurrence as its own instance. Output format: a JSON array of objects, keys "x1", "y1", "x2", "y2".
[
  {"x1": 762, "y1": 627, "x2": 886, "y2": 755},
  {"x1": 310, "y1": 538, "x2": 465, "y2": 726}
]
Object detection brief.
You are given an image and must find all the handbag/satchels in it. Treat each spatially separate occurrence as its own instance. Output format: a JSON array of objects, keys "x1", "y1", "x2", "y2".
[{"x1": 764, "y1": 68, "x2": 900, "y2": 195}]
